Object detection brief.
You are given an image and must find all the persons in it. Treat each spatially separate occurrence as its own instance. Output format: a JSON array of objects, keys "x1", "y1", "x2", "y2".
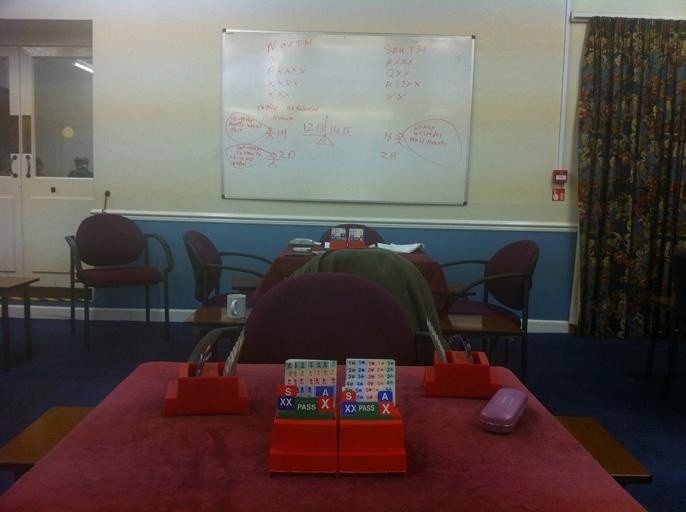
[
  {"x1": 66, "y1": 152, "x2": 93, "y2": 178},
  {"x1": 0, "y1": 158, "x2": 12, "y2": 176},
  {"x1": 35, "y1": 158, "x2": 45, "y2": 176}
]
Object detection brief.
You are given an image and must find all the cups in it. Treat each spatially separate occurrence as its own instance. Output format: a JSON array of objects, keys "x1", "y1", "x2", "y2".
[{"x1": 227, "y1": 294, "x2": 246, "y2": 319}]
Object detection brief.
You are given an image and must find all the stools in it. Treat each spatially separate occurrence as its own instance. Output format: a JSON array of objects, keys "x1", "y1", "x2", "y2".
[
  {"x1": 0, "y1": 405, "x2": 96, "y2": 481},
  {"x1": 554, "y1": 414, "x2": 652, "y2": 489}
]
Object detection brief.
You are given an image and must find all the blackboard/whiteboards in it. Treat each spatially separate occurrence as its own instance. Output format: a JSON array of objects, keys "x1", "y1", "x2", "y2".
[{"x1": 221, "y1": 28, "x2": 475, "y2": 206}]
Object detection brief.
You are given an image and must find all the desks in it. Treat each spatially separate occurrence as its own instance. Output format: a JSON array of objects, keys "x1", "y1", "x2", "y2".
[
  {"x1": 1, "y1": 276, "x2": 40, "y2": 365},
  {"x1": 0, "y1": 357, "x2": 647, "y2": 512}
]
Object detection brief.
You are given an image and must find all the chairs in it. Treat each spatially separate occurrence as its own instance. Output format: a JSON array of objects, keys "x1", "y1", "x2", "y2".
[
  {"x1": 185, "y1": 225, "x2": 539, "y2": 377},
  {"x1": 63, "y1": 213, "x2": 175, "y2": 351}
]
